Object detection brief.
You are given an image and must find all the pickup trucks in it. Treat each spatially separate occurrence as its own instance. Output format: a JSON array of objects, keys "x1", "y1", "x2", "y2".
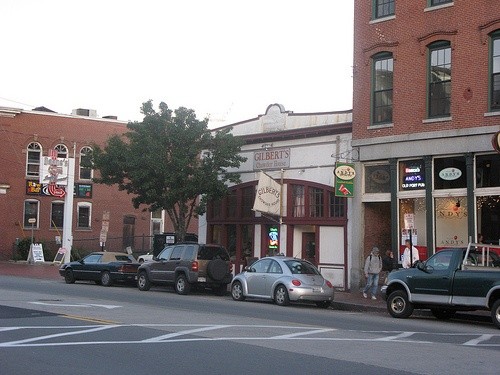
[{"x1": 381, "y1": 236, "x2": 500, "y2": 329}]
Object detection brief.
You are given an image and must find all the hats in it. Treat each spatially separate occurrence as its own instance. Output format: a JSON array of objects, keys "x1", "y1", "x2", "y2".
[{"x1": 372, "y1": 247, "x2": 379, "y2": 253}]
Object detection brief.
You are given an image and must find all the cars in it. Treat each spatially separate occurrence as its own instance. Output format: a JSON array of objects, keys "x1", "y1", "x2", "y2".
[{"x1": 59, "y1": 252, "x2": 142, "y2": 287}]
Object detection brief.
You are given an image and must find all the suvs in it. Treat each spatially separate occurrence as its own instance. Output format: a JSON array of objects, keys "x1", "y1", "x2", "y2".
[{"x1": 136, "y1": 241, "x2": 234, "y2": 296}]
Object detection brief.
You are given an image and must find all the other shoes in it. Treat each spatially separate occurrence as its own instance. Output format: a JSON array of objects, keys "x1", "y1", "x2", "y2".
[
  {"x1": 372, "y1": 296, "x2": 376, "y2": 299},
  {"x1": 363, "y1": 293, "x2": 367, "y2": 298}
]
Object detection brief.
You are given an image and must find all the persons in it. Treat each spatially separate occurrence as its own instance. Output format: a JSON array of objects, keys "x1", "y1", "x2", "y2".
[
  {"x1": 401, "y1": 239, "x2": 419, "y2": 269},
  {"x1": 361, "y1": 247, "x2": 382, "y2": 300},
  {"x1": 477, "y1": 232, "x2": 485, "y2": 245},
  {"x1": 382, "y1": 249, "x2": 395, "y2": 282}
]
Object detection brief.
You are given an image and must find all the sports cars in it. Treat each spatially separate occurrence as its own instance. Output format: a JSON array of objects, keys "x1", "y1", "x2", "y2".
[{"x1": 230, "y1": 253, "x2": 334, "y2": 308}]
